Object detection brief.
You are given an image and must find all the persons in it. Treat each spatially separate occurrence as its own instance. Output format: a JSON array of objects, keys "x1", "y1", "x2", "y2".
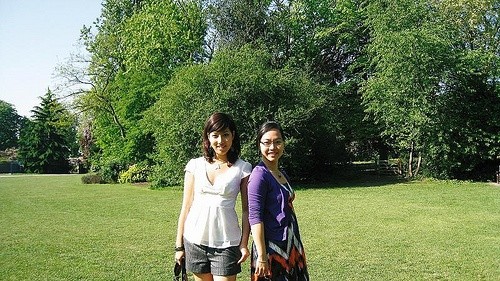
[
  {"x1": 173, "y1": 112, "x2": 253, "y2": 281},
  {"x1": 247, "y1": 121, "x2": 310, "y2": 281}
]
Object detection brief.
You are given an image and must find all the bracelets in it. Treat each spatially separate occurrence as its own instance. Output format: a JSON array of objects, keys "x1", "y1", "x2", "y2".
[
  {"x1": 174, "y1": 245, "x2": 184, "y2": 251},
  {"x1": 259, "y1": 260, "x2": 267, "y2": 263}
]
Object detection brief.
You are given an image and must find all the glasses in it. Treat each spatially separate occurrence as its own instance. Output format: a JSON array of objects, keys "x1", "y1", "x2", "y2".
[{"x1": 259, "y1": 140, "x2": 284, "y2": 146}]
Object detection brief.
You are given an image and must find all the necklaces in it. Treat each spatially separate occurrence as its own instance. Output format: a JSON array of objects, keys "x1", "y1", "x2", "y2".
[
  {"x1": 214, "y1": 160, "x2": 227, "y2": 170},
  {"x1": 276, "y1": 172, "x2": 282, "y2": 178}
]
